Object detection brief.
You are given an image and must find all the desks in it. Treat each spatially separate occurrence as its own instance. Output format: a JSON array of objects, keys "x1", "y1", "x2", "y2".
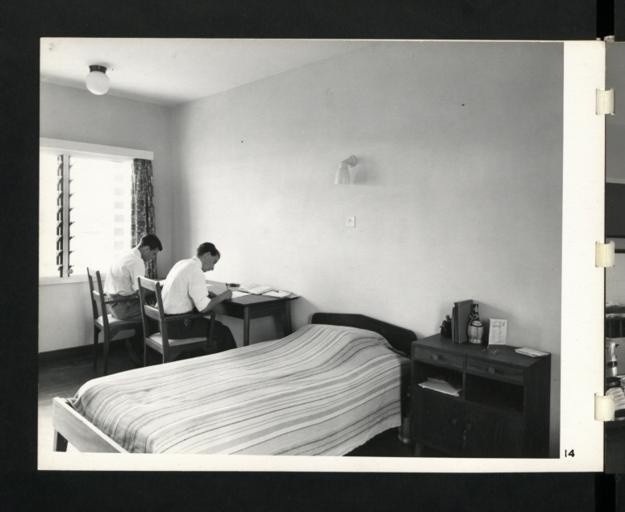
[{"x1": 151, "y1": 278, "x2": 301, "y2": 347}]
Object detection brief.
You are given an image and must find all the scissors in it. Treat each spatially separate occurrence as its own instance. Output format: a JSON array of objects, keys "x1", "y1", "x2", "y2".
[{"x1": 468, "y1": 312, "x2": 479, "y2": 325}]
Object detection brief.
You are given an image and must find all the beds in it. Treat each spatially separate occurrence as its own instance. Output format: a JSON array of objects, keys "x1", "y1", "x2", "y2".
[{"x1": 50, "y1": 312, "x2": 417, "y2": 454}]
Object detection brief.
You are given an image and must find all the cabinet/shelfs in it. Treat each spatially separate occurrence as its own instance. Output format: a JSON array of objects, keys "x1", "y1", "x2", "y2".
[{"x1": 411, "y1": 334, "x2": 551, "y2": 457}]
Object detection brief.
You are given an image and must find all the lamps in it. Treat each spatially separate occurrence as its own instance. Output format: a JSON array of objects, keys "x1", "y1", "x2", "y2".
[{"x1": 84, "y1": 65, "x2": 112, "y2": 96}]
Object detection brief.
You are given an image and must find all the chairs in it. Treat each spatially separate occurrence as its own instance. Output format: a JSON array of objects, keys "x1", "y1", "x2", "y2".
[{"x1": 87, "y1": 267, "x2": 223, "y2": 376}]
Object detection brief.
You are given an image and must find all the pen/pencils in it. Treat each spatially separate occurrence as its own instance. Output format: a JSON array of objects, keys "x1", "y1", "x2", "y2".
[{"x1": 226, "y1": 284, "x2": 230, "y2": 291}]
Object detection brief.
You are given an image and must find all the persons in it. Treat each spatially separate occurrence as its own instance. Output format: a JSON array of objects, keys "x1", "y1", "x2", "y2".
[
  {"x1": 104, "y1": 233, "x2": 163, "y2": 363},
  {"x1": 154, "y1": 243, "x2": 237, "y2": 352}
]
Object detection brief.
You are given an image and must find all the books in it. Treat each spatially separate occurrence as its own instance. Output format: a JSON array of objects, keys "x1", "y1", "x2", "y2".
[
  {"x1": 417, "y1": 372, "x2": 461, "y2": 398},
  {"x1": 232, "y1": 284, "x2": 293, "y2": 300}
]
